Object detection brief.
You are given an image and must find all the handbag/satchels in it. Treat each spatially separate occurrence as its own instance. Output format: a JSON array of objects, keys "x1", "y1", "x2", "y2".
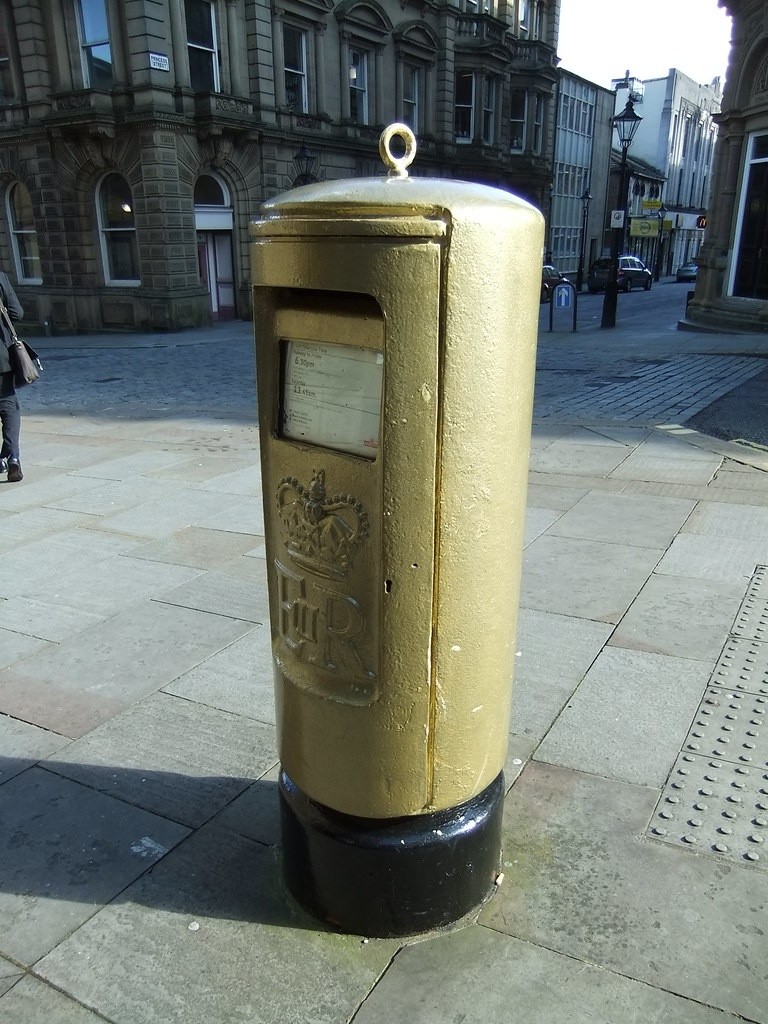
[{"x1": 0, "y1": 298, "x2": 43, "y2": 389}]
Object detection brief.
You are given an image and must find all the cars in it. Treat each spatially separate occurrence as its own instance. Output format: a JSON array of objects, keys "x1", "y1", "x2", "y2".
[
  {"x1": 588, "y1": 255, "x2": 653, "y2": 294},
  {"x1": 542, "y1": 266, "x2": 567, "y2": 306},
  {"x1": 676, "y1": 262, "x2": 698, "y2": 282}
]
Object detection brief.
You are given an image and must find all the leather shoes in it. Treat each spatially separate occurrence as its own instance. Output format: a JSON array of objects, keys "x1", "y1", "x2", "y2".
[{"x1": 7, "y1": 457, "x2": 23, "y2": 482}]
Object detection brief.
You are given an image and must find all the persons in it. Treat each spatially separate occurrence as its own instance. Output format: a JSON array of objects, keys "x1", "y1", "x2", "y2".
[{"x1": 0, "y1": 271, "x2": 25, "y2": 482}]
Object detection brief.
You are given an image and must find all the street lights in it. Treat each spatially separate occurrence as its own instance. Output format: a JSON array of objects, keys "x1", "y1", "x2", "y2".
[{"x1": 599, "y1": 98, "x2": 644, "y2": 329}]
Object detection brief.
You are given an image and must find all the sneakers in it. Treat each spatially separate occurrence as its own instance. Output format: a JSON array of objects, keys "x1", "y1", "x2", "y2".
[{"x1": 0, "y1": 457, "x2": 8, "y2": 472}]
